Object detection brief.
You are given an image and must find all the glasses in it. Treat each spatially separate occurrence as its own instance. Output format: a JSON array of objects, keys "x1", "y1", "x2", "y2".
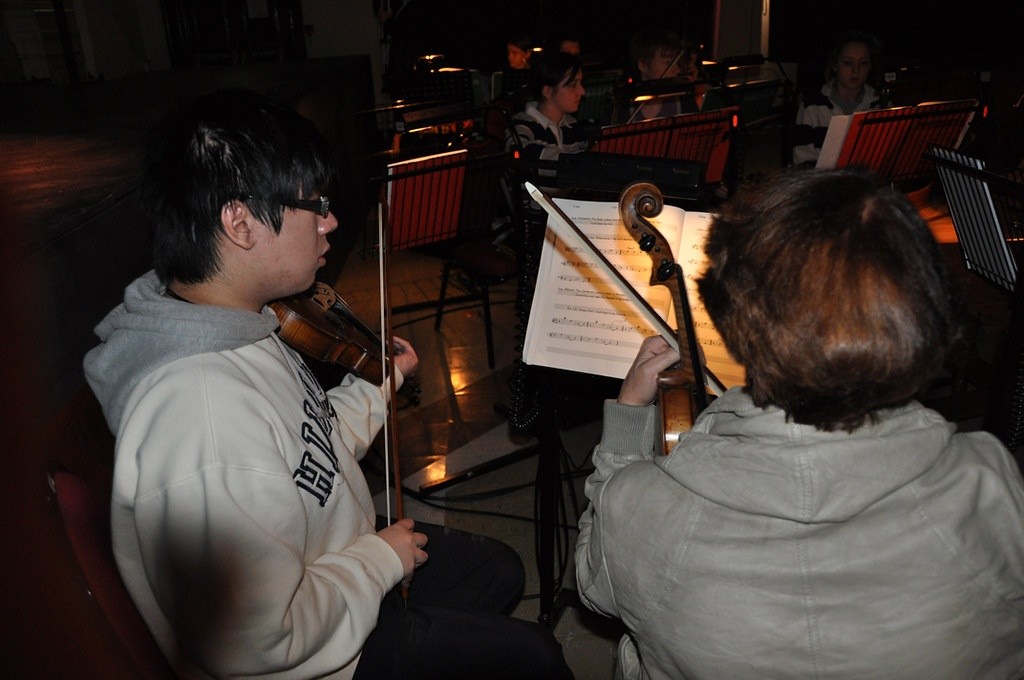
[{"x1": 230, "y1": 186, "x2": 331, "y2": 218}]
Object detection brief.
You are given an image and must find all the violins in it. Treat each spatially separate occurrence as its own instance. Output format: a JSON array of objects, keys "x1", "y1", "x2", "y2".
[
  {"x1": 619, "y1": 179, "x2": 710, "y2": 456},
  {"x1": 267, "y1": 281, "x2": 422, "y2": 406}
]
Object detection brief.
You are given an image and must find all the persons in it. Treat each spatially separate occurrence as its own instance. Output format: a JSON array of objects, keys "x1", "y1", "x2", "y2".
[
  {"x1": 84, "y1": 83, "x2": 576, "y2": 680},
  {"x1": 575, "y1": 167, "x2": 1024, "y2": 680},
  {"x1": 500, "y1": 32, "x2": 895, "y2": 285}
]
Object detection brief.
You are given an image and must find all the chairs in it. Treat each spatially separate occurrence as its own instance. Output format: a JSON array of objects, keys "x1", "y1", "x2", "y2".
[
  {"x1": 46, "y1": 468, "x2": 176, "y2": 680},
  {"x1": 434, "y1": 95, "x2": 518, "y2": 370}
]
